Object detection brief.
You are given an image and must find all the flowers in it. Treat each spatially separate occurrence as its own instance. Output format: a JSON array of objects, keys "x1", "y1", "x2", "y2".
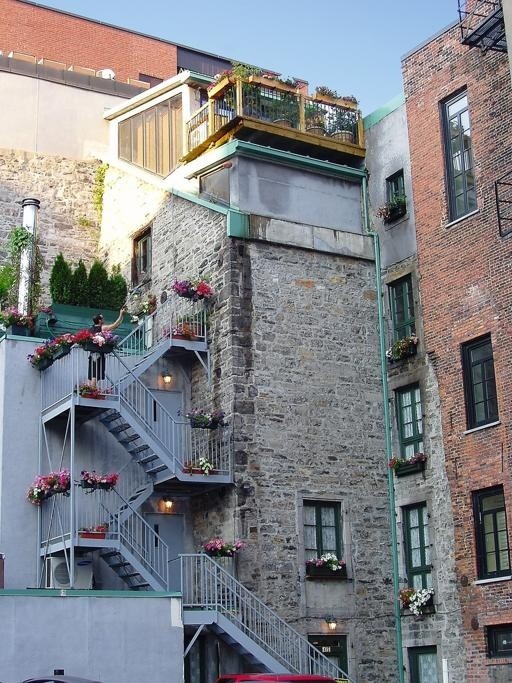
[
  {"x1": 162, "y1": 310, "x2": 210, "y2": 340},
  {"x1": 389, "y1": 452, "x2": 425, "y2": 469},
  {"x1": 399, "y1": 586, "x2": 433, "y2": 617},
  {"x1": 74, "y1": 381, "x2": 111, "y2": 397},
  {"x1": 77, "y1": 522, "x2": 109, "y2": 534},
  {"x1": 176, "y1": 408, "x2": 227, "y2": 430},
  {"x1": 193, "y1": 538, "x2": 247, "y2": 558},
  {"x1": 184, "y1": 456, "x2": 215, "y2": 477},
  {"x1": 374, "y1": 195, "x2": 405, "y2": 222},
  {"x1": 304, "y1": 550, "x2": 345, "y2": 575},
  {"x1": 25, "y1": 329, "x2": 120, "y2": 370},
  {"x1": 24, "y1": 469, "x2": 119, "y2": 504},
  {"x1": 173, "y1": 273, "x2": 214, "y2": 301},
  {"x1": 385, "y1": 336, "x2": 417, "y2": 361}
]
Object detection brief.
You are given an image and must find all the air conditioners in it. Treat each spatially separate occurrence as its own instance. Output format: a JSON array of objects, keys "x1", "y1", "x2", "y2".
[{"x1": 45, "y1": 557, "x2": 94, "y2": 590}]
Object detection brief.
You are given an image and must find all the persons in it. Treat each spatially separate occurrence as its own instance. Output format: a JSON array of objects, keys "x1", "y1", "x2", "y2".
[{"x1": 87, "y1": 306, "x2": 127, "y2": 390}]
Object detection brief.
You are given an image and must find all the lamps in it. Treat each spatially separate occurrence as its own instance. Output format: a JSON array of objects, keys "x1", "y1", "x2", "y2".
[
  {"x1": 164, "y1": 495, "x2": 174, "y2": 508},
  {"x1": 326, "y1": 613, "x2": 337, "y2": 630},
  {"x1": 163, "y1": 370, "x2": 172, "y2": 383}
]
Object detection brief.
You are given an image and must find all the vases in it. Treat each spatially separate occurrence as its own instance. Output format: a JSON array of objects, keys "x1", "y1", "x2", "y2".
[
  {"x1": 81, "y1": 393, "x2": 105, "y2": 401},
  {"x1": 385, "y1": 207, "x2": 406, "y2": 223},
  {"x1": 396, "y1": 461, "x2": 426, "y2": 476},
  {"x1": 307, "y1": 565, "x2": 347, "y2": 580},
  {"x1": 80, "y1": 532, "x2": 105, "y2": 539}
]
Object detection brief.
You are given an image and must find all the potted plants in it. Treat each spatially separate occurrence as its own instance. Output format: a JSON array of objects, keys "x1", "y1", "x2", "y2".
[{"x1": 269, "y1": 102, "x2": 356, "y2": 146}]
